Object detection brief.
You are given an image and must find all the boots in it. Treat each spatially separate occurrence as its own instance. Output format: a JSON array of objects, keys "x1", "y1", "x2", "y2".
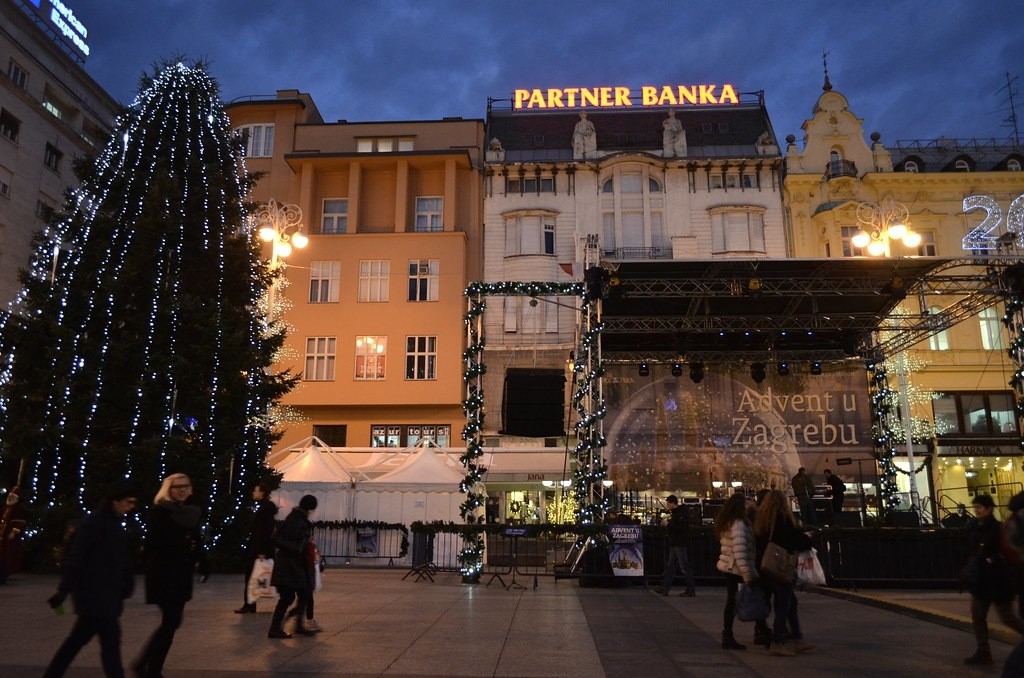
[
  {"x1": 722, "y1": 630, "x2": 746, "y2": 650},
  {"x1": 754, "y1": 626, "x2": 772, "y2": 646}
]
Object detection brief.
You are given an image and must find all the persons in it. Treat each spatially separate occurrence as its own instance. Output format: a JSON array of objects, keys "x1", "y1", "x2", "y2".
[
  {"x1": 714, "y1": 487, "x2": 811, "y2": 656},
  {"x1": 823, "y1": 468, "x2": 846, "y2": 526},
  {"x1": 135, "y1": 473, "x2": 212, "y2": 678},
  {"x1": 234, "y1": 482, "x2": 323, "y2": 639},
  {"x1": 961, "y1": 491, "x2": 1024, "y2": 672},
  {"x1": 573, "y1": 110, "x2": 594, "y2": 134},
  {"x1": 792, "y1": 467, "x2": 816, "y2": 526},
  {"x1": 663, "y1": 109, "x2": 683, "y2": 131},
  {"x1": 0, "y1": 485, "x2": 29, "y2": 580},
  {"x1": 45, "y1": 478, "x2": 141, "y2": 678},
  {"x1": 647, "y1": 495, "x2": 696, "y2": 597}
]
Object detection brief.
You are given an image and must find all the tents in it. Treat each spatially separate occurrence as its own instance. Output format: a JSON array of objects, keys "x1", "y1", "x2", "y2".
[{"x1": 259, "y1": 436, "x2": 488, "y2": 567}]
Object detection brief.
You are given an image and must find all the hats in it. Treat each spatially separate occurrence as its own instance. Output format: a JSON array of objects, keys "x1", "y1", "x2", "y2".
[
  {"x1": 300, "y1": 495, "x2": 317, "y2": 510},
  {"x1": 973, "y1": 495, "x2": 992, "y2": 506}
]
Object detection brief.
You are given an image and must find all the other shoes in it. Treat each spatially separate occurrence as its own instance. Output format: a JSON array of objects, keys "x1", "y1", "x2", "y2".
[
  {"x1": 266, "y1": 626, "x2": 292, "y2": 639},
  {"x1": 655, "y1": 586, "x2": 668, "y2": 596},
  {"x1": 965, "y1": 648, "x2": 994, "y2": 666},
  {"x1": 235, "y1": 603, "x2": 257, "y2": 614},
  {"x1": 295, "y1": 620, "x2": 323, "y2": 635},
  {"x1": 680, "y1": 589, "x2": 696, "y2": 597},
  {"x1": 770, "y1": 640, "x2": 816, "y2": 657}
]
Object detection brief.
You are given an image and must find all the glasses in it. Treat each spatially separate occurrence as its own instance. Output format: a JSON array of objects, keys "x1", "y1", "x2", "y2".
[
  {"x1": 125, "y1": 497, "x2": 134, "y2": 505},
  {"x1": 172, "y1": 484, "x2": 191, "y2": 489}
]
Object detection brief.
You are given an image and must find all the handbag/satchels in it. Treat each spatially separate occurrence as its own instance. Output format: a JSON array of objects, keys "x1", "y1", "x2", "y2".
[
  {"x1": 736, "y1": 583, "x2": 766, "y2": 621},
  {"x1": 269, "y1": 514, "x2": 309, "y2": 555},
  {"x1": 246, "y1": 558, "x2": 274, "y2": 604},
  {"x1": 761, "y1": 544, "x2": 796, "y2": 586},
  {"x1": 797, "y1": 549, "x2": 826, "y2": 587}
]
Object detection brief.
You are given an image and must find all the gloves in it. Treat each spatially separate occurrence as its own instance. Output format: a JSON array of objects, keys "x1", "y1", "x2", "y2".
[{"x1": 48, "y1": 592, "x2": 67, "y2": 607}]
